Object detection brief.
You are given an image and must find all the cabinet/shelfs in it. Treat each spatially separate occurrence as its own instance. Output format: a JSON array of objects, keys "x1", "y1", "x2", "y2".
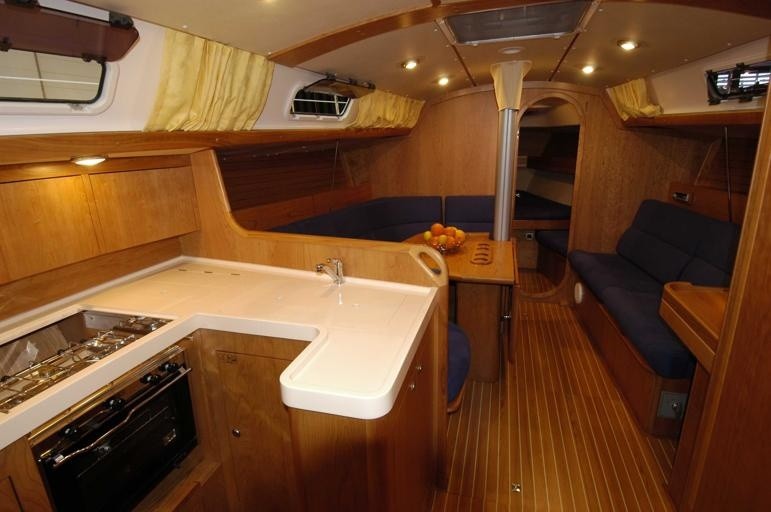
[
  {"x1": 401, "y1": 232, "x2": 520, "y2": 383},
  {"x1": 197, "y1": 328, "x2": 434, "y2": 512}
]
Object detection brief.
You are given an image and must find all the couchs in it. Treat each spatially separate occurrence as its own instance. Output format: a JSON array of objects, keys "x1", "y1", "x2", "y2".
[
  {"x1": 266, "y1": 196, "x2": 497, "y2": 413},
  {"x1": 568, "y1": 199, "x2": 742, "y2": 439}
]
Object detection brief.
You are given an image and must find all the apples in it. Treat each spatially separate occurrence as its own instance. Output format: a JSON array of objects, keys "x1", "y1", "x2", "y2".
[{"x1": 423, "y1": 224, "x2": 466, "y2": 248}]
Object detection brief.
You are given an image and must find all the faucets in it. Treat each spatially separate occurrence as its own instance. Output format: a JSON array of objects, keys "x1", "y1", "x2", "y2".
[{"x1": 316, "y1": 255, "x2": 347, "y2": 286}]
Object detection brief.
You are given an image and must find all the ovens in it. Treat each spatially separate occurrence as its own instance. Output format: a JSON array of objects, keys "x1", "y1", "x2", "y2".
[{"x1": 25, "y1": 343, "x2": 202, "y2": 512}]
